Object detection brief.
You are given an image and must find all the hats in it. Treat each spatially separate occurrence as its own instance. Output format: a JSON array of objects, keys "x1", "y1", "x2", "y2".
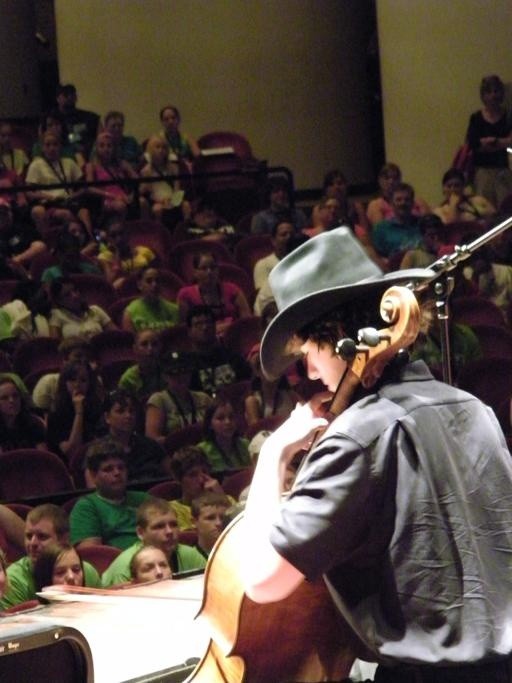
[{"x1": 259, "y1": 226, "x2": 436, "y2": 382}]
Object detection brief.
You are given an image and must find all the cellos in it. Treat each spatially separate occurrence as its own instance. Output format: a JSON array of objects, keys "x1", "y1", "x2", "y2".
[{"x1": 182, "y1": 284, "x2": 422, "y2": 683}]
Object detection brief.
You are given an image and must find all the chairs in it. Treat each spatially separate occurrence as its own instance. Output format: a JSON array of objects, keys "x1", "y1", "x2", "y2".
[
  {"x1": 2, "y1": 120, "x2": 510, "y2": 594},
  {"x1": 0, "y1": 622, "x2": 94, "y2": 681}
]
